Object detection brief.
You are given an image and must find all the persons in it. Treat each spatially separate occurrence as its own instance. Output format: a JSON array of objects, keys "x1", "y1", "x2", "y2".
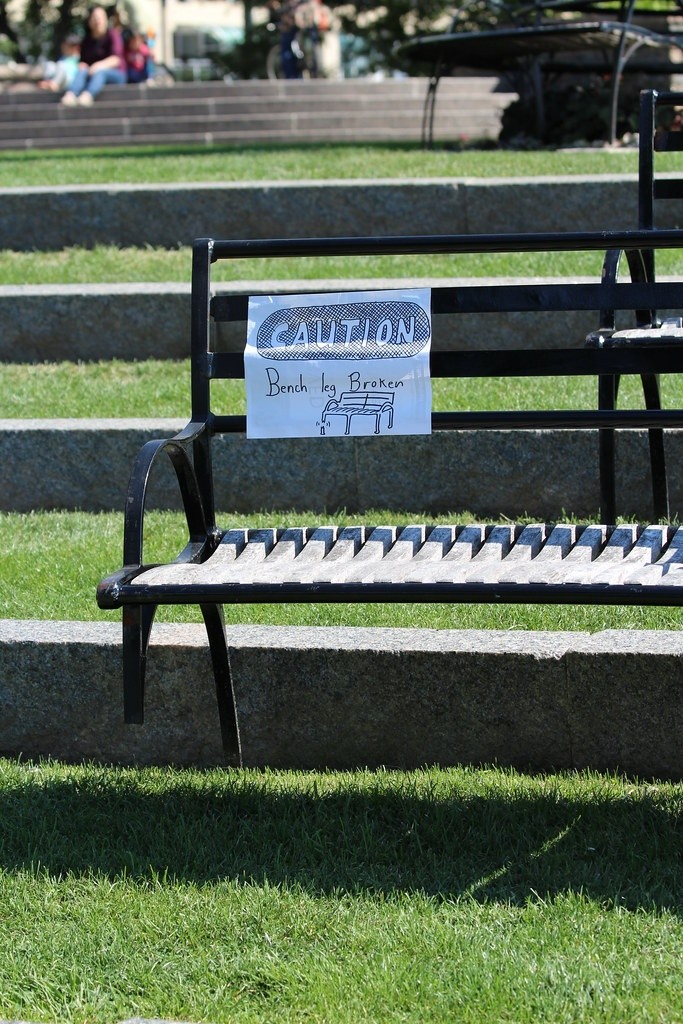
[
  {"x1": 275, "y1": 0, "x2": 343, "y2": 79},
  {"x1": 39, "y1": 6, "x2": 153, "y2": 105}
]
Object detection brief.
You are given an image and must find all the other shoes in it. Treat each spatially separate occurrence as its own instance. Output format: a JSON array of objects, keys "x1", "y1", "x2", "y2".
[
  {"x1": 39, "y1": 80, "x2": 50, "y2": 91},
  {"x1": 76, "y1": 91, "x2": 93, "y2": 107},
  {"x1": 50, "y1": 81, "x2": 59, "y2": 93},
  {"x1": 60, "y1": 91, "x2": 76, "y2": 105}
]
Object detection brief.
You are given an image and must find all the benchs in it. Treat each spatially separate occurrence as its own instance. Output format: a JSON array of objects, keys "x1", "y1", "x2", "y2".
[
  {"x1": 392, "y1": 0, "x2": 683, "y2": 148},
  {"x1": 585, "y1": 90, "x2": 683, "y2": 525},
  {"x1": 97, "y1": 229, "x2": 683, "y2": 769}
]
